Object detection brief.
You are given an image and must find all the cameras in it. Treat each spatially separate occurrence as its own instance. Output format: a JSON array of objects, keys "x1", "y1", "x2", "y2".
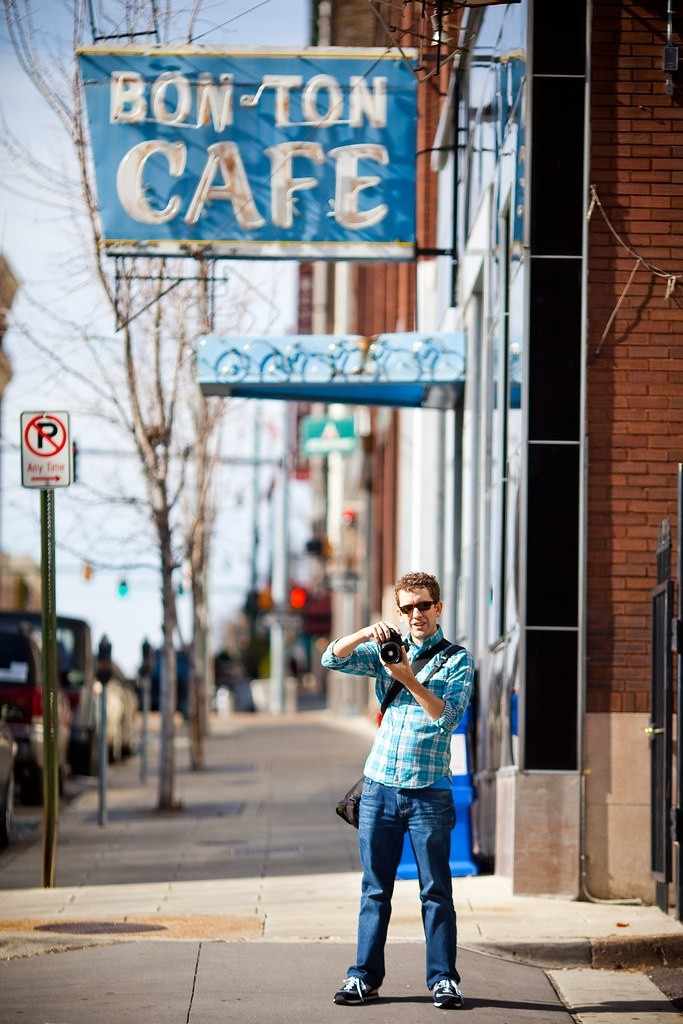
[{"x1": 380, "y1": 628, "x2": 409, "y2": 664}]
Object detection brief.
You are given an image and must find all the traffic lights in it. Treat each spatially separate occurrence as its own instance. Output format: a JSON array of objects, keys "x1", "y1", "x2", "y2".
[
  {"x1": 289, "y1": 587, "x2": 308, "y2": 610},
  {"x1": 306, "y1": 538, "x2": 322, "y2": 555}
]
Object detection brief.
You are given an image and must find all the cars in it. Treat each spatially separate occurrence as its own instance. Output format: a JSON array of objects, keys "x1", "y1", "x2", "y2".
[{"x1": 1, "y1": 609, "x2": 139, "y2": 850}]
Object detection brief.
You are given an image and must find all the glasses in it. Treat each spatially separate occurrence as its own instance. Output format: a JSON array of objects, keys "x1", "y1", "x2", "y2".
[{"x1": 398, "y1": 600, "x2": 434, "y2": 614}]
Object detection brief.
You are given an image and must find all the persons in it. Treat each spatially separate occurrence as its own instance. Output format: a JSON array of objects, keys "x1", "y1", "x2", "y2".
[{"x1": 321, "y1": 572, "x2": 476, "y2": 1008}]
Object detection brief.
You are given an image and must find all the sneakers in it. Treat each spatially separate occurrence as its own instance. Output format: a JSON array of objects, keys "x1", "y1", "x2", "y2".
[
  {"x1": 332, "y1": 976, "x2": 380, "y2": 1006},
  {"x1": 431, "y1": 978, "x2": 465, "y2": 1009}
]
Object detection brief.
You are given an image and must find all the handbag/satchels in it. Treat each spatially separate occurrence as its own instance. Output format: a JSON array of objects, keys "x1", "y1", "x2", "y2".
[{"x1": 334, "y1": 776, "x2": 365, "y2": 830}]
